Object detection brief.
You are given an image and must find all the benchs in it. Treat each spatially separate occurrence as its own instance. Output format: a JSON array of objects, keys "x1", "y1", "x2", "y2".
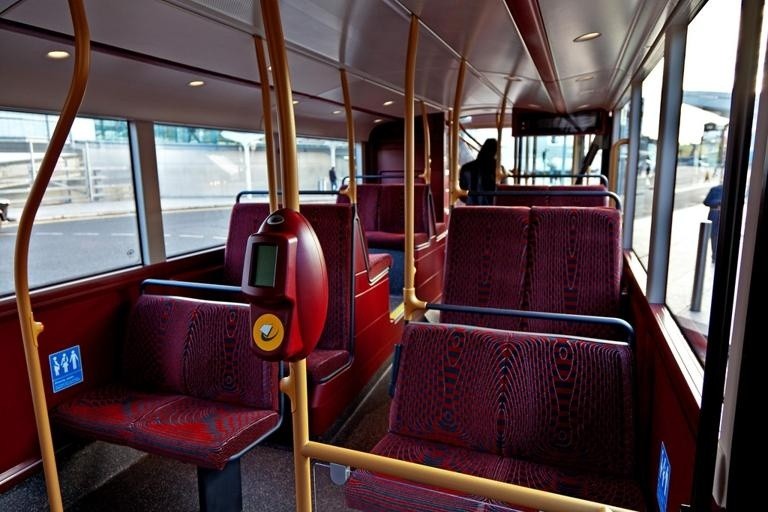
[
  {"x1": 335, "y1": 185, "x2": 429, "y2": 248},
  {"x1": 429, "y1": 190, "x2": 446, "y2": 237},
  {"x1": 222, "y1": 204, "x2": 354, "y2": 384},
  {"x1": 50, "y1": 276, "x2": 284, "y2": 511},
  {"x1": 356, "y1": 216, "x2": 393, "y2": 280},
  {"x1": 441, "y1": 204, "x2": 624, "y2": 342},
  {"x1": 343, "y1": 321, "x2": 648, "y2": 510},
  {"x1": 496, "y1": 182, "x2": 609, "y2": 208}
]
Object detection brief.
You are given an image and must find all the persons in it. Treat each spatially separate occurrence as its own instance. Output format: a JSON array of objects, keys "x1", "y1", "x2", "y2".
[
  {"x1": 329, "y1": 167, "x2": 337, "y2": 191},
  {"x1": 467, "y1": 138, "x2": 508, "y2": 205},
  {"x1": 703, "y1": 185, "x2": 723, "y2": 264}
]
